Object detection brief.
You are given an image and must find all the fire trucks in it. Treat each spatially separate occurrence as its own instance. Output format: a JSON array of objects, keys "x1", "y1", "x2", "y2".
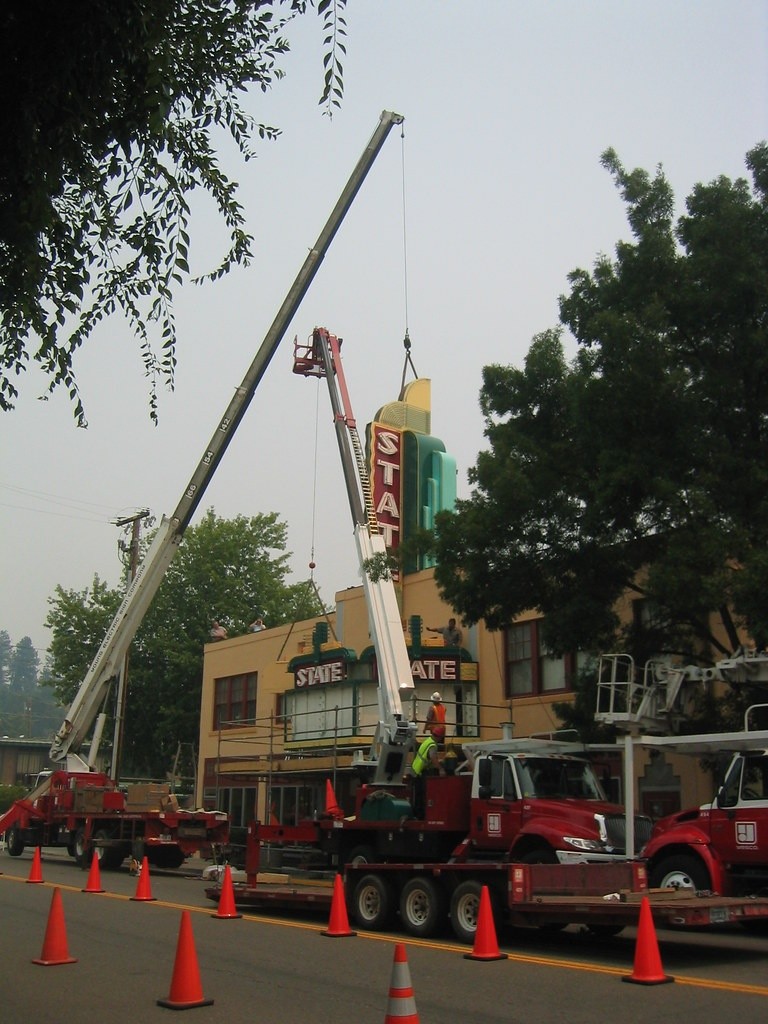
[
  {"x1": 207, "y1": 322, "x2": 768, "y2": 944},
  {"x1": 0, "y1": 103, "x2": 410, "y2": 870}
]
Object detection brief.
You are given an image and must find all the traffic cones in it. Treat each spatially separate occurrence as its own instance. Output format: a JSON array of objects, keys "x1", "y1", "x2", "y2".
[
  {"x1": 154, "y1": 909, "x2": 214, "y2": 1011},
  {"x1": 619, "y1": 896, "x2": 676, "y2": 985},
  {"x1": 211, "y1": 864, "x2": 244, "y2": 919},
  {"x1": 320, "y1": 873, "x2": 357, "y2": 936},
  {"x1": 26, "y1": 846, "x2": 44, "y2": 883},
  {"x1": 381, "y1": 943, "x2": 420, "y2": 1024},
  {"x1": 81, "y1": 850, "x2": 106, "y2": 893},
  {"x1": 125, "y1": 856, "x2": 159, "y2": 902},
  {"x1": 463, "y1": 885, "x2": 509, "y2": 961},
  {"x1": 32, "y1": 886, "x2": 78, "y2": 966}
]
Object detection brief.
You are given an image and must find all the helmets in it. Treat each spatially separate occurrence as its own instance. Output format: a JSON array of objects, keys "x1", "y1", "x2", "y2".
[
  {"x1": 431, "y1": 692, "x2": 442, "y2": 701},
  {"x1": 432, "y1": 726, "x2": 445, "y2": 736}
]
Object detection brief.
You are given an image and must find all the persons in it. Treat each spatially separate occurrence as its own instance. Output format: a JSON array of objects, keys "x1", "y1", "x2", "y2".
[
  {"x1": 210, "y1": 620, "x2": 228, "y2": 643},
  {"x1": 426, "y1": 619, "x2": 463, "y2": 647},
  {"x1": 409, "y1": 726, "x2": 445, "y2": 821},
  {"x1": 249, "y1": 617, "x2": 267, "y2": 633},
  {"x1": 423, "y1": 691, "x2": 447, "y2": 751}
]
